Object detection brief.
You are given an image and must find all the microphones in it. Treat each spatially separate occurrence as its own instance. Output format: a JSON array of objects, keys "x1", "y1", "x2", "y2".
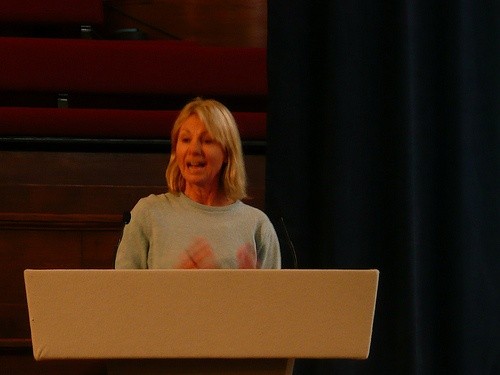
[
  {"x1": 278, "y1": 214, "x2": 298, "y2": 267},
  {"x1": 113, "y1": 211, "x2": 131, "y2": 269}
]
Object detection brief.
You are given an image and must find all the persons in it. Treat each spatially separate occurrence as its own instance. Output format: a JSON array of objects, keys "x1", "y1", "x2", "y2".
[{"x1": 113, "y1": 98, "x2": 283, "y2": 269}]
